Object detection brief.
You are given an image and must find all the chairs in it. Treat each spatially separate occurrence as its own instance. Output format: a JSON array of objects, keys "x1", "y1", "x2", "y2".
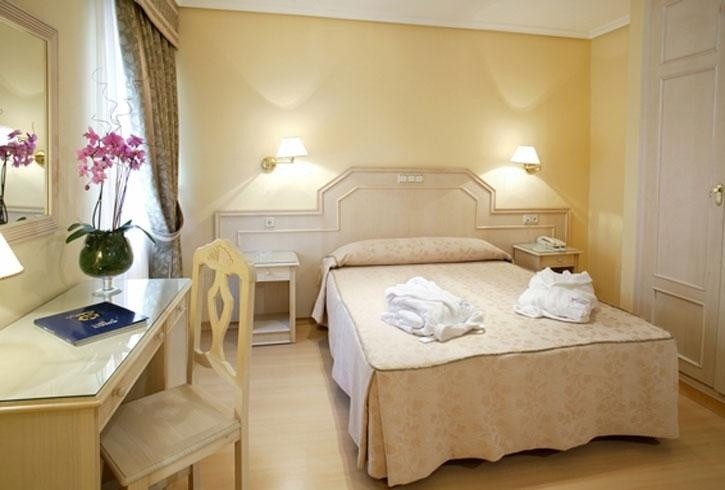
[{"x1": 100, "y1": 238, "x2": 256, "y2": 489}]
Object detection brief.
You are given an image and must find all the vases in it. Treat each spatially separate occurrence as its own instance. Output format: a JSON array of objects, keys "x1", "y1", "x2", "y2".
[{"x1": 78, "y1": 226, "x2": 137, "y2": 298}]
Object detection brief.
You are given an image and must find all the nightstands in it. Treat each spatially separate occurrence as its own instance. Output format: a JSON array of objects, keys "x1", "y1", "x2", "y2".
[
  {"x1": 514, "y1": 241, "x2": 584, "y2": 276},
  {"x1": 240, "y1": 250, "x2": 301, "y2": 345}
]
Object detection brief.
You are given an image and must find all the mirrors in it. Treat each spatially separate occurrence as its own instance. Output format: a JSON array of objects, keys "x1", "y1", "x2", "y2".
[{"x1": 0, "y1": 3, "x2": 59, "y2": 249}]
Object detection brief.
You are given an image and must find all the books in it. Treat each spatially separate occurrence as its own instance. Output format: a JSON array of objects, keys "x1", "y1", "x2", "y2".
[{"x1": 30, "y1": 302, "x2": 151, "y2": 348}]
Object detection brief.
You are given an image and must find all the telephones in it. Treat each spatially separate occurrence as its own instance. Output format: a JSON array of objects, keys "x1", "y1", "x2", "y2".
[{"x1": 537, "y1": 236, "x2": 567, "y2": 248}]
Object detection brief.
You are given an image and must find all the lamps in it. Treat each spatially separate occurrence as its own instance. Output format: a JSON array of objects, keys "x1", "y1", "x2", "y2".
[
  {"x1": 261, "y1": 138, "x2": 309, "y2": 175},
  {"x1": 511, "y1": 145, "x2": 543, "y2": 175}
]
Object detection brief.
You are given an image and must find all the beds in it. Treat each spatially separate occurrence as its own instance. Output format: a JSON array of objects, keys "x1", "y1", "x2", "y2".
[{"x1": 309, "y1": 164, "x2": 680, "y2": 490}]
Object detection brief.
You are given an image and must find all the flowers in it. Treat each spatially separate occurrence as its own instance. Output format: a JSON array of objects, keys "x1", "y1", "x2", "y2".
[
  {"x1": 1, "y1": 130, "x2": 40, "y2": 170},
  {"x1": 67, "y1": 127, "x2": 149, "y2": 230}
]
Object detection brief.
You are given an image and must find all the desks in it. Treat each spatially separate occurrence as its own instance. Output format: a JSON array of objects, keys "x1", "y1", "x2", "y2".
[{"x1": 2, "y1": 273, "x2": 196, "y2": 490}]
